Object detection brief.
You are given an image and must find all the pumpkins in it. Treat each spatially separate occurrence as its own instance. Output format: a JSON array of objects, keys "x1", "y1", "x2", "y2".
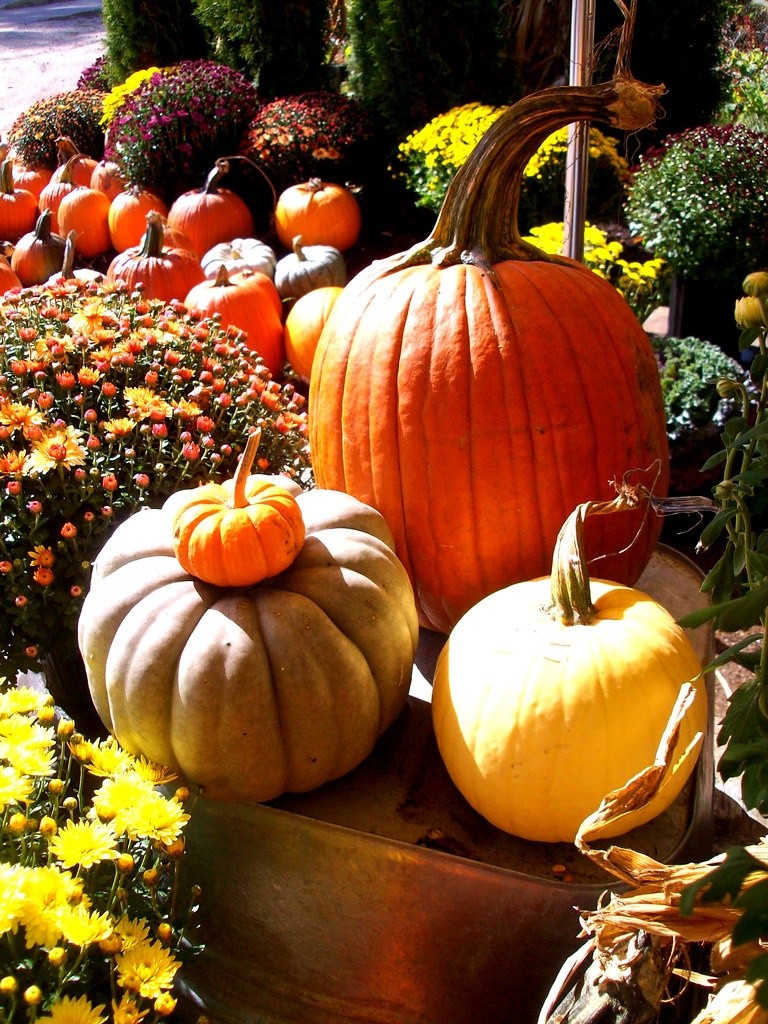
[
  {"x1": 77, "y1": 473, "x2": 419, "y2": 807},
  {"x1": 433, "y1": 501, "x2": 707, "y2": 842},
  {"x1": 172, "y1": 428, "x2": 305, "y2": 585},
  {"x1": 0, "y1": 139, "x2": 358, "y2": 383},
  {"x1": 309, "y1": 78, "x2": 670, "y2": 628}
]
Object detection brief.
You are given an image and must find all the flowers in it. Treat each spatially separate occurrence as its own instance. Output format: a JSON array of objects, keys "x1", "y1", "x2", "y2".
[
  {"x1": 99, "y1": 57, "x2": 261, "y2": 195},
  {"x1": 2, "y1": 684, "x2": 210, "y2": 1024},
  {"x1": 75, "y1": 53, "x2": 110, "y2": 96},
  {"x1": 390, "y1": 93, "x2": 626, "y2": 244},
  {"x1": 3, "y1": 269, "x2": 308, "y2": 653},
  {"x1": 237, "y1": 89, "x2": 374, "y2": 184},
  {"x1": 4, "y1": 88, "x2": 110, "y2": 170},
  {"x1": 97, "y1": 65, "x2": 170, "y2": 130},
  {"x1": 521, "y1": 220, "x2": 675, "y2": 333},
  {"x1": 624, "y1": 123, "x2": 768, "y2": 288}
]
